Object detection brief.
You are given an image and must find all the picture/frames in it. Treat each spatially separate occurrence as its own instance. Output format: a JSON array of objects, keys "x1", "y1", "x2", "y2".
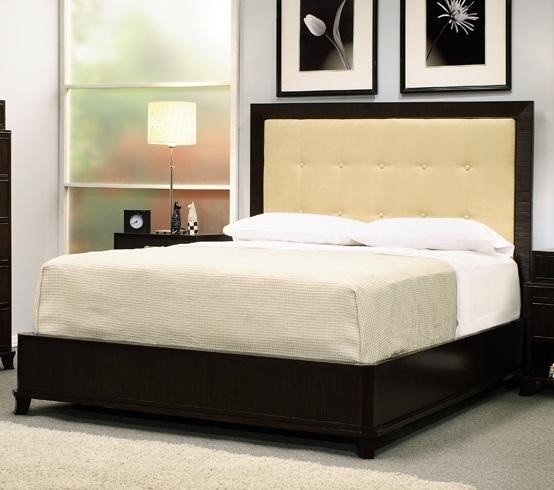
[{"x1": 276, "y1": 0, "x2": 513, "y2": 98}]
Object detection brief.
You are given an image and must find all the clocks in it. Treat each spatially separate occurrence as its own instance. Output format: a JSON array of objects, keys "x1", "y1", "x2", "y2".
[{"x1": 124, "y1": 210, "x2": 151, "y2": 233}]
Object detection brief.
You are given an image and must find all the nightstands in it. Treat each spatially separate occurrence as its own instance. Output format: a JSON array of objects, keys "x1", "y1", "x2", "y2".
[
  {"x1": 114, "y1": 232, "x2": 232, "y2": 249},
  {"x1": 516, "y1": 276, "x2": 553, "y2": 398}
]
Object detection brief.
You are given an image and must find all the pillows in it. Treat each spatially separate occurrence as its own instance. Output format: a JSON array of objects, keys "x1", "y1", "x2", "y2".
[{"x1": 222, "y1": 212, "x2": 513, "y2": 256}]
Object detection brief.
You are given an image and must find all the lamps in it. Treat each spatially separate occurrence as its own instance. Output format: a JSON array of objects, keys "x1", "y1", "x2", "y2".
[{"x1": 147, "y1": 99, "x2": 197, "y2": 234}]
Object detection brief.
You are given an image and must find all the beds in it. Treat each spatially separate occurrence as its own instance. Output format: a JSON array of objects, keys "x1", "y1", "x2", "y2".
[{"x1": 10, "y1": 101, "x2": 534, "y2": 460}]
